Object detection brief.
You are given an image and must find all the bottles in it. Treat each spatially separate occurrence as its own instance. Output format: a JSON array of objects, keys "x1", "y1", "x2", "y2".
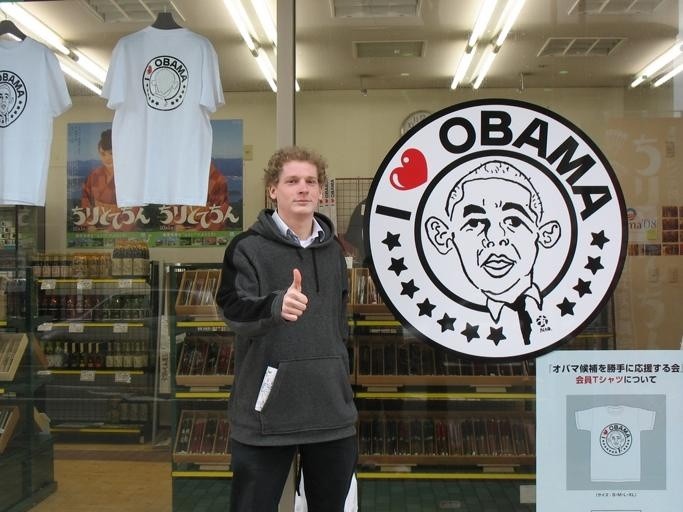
[{"x1": 0, "y1": 240, "x2": 152, "y2": 430}]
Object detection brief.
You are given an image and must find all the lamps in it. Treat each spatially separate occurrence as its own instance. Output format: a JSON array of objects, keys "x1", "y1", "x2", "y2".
[
  {"x1": 630, "y1": 37, "x2": 683, "y2": 90},
  {"x1": 451, "y1": 0, "x2": 525, "y2": 92},
  {"x1": 0, "y1": 0, "x2": 110, "y2": 100},
  {"x1": 222, "y1": 0, "x2": 302, "y2": 95}
]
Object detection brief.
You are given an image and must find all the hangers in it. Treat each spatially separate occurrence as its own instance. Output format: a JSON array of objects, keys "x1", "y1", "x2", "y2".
[{"x1": 150, "y1": 0, "x2": 183, "y2": 29}]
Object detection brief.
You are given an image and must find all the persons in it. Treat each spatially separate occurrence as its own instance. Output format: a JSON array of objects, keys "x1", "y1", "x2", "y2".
[
  {"x1": 82, "y1": 130, "x2": 142, "y2": 232},
  {"x1": 215, "y1": 145, "x2": 359, "y2": 510},
  {"x1": 424, "y1": 159, "x2": 561, "y2": 321}
]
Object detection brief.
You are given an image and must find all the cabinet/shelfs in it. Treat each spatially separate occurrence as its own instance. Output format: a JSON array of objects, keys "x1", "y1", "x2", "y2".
[
  {"x1": 30, "y1": 253, "x2": 158, "y2": 444},
  {"x1": 172, "y1": 258, "x2": 618, "y2": 478},
  {"x1": 0, "y1": 201, "x2": 58, "y2": 512}
]
